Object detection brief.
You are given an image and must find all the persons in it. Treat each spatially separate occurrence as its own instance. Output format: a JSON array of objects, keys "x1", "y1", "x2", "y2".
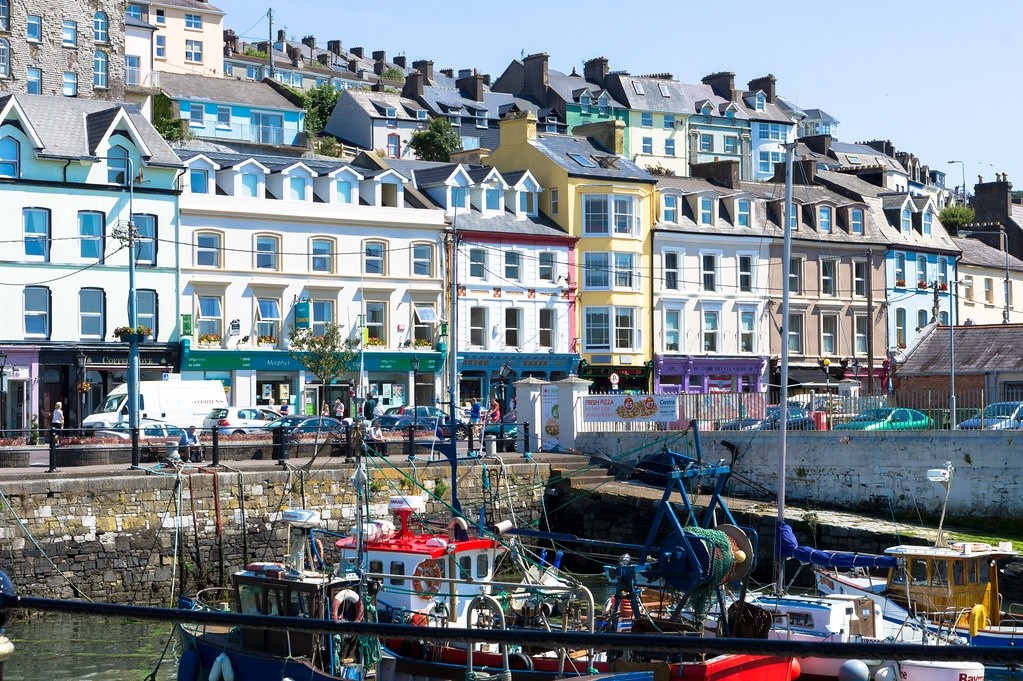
[
  {"x1": 333, "y1": 397, "x2": 344, "y2": 422},
  {"x1": 323, "y1": 404, "x2": 330, "y2": 417},
  {"x1": 364, "y1": 393, "x2": 376, "y2": 420},
  {"x1": 51, "y1": 402, "x2": 64, "y2": 440},
  {"x1": 353, "y1": 426, "x2": 362, "y2": 457},
  {"x1": 373, "y1": 402, "x2": 386, "y2": 418},
  {"x1": 280, "y1": 400, "x2": 289, "y2": 416},
  {"x1": 267, "y1": 398, "x2": 276, "y2": 421},
  {"x1": 470, "y1": 398, "x2": 481, "y2": 438},
  {"x1": 178, "y1": 425, "x2": 205, "y2": 463},
  {"x1": 369, "y1": 423, "x2": 389, "y2": 457},
  {"x1": 489, "y1": 396, "x2": 500, "y2": 423}
]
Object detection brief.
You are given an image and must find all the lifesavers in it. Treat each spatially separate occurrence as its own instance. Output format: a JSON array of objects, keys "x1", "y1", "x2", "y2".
[
  {"x1": 968, "y1": 604, "x2": 986, "y2": 636},
  {"x1": 246, "y1": 561, "x2": 282, "y2": 572},
  {"x1": 306, "y1": 540, "x2": 324, "y2": 565},
  {"x1": 331, "y1": 589, "x2": 366, "y2": 623},
  {"x1": 413, "y1": 561, "x2": 444, "y2": 599}
]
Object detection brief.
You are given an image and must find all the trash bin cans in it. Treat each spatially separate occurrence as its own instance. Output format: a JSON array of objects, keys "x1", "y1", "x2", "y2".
[
  {"x1": 272, "y1": 428, "x2": 289, "y2": 460},
  {"x1": 814, "y1": 411, "x2": 826, "y2": 431}
]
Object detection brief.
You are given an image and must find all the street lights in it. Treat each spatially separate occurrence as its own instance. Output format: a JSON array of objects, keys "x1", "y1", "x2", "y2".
[
  {"x1": 0, "y1": 349, "x2": 7, "y2": 439},
  {"x1": 76, "y1": 351, "x2": 86, "y2": 439},
  {"x1": 449, "y1": 178, "x2": 500, "y2": 466},
  {"x1": 949, "y1": 279, "x2": 972, "y2": 430},
  {"x1": 824, "y1": 358, "x2": 830, "y2": 414},
  {"x1": 499, "y1": 360, "x2": 513, "y2": 450},
  {"x1": 408, "y1": 354, "x2": 420, "y2": 461},
  {"x1": 78, "y1": 155, "x2": 139, "y2": 471}
]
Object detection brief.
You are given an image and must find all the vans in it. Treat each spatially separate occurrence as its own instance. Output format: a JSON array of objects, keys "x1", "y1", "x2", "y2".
[{"x1": 78, "y1": 379, "x2": 229, "y2": 433}]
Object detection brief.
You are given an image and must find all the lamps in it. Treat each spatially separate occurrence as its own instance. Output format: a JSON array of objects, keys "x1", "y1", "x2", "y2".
[
  {"x1": 13, "y1": 365, "x2": 19, "y2": 371},
  {"x1": 134, "y1": 168, "x2": 144, "y2": 184}
]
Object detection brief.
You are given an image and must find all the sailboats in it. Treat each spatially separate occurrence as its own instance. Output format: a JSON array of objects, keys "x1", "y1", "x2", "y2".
[{"x1": 157, "y1": 139, "x2": 1023, "y2": 681}]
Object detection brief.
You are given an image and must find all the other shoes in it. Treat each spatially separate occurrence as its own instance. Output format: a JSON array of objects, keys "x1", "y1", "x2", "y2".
[
  {"x1": 371, "y1": 454, "x2": 377, "y2": 457},
  {"x1": 187, "y1": 459, "x2": 192, "y2": 463},
  {"x1": 383, "y1": 452, "x2": 389, "y2": 456},
  {"x1": 202, "y1": 460, "x2": 205, "y2": 463}
]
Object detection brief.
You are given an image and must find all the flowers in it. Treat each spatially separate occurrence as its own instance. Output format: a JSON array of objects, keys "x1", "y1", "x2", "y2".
[
  {"x1": 259, "y1": 335, "x2": 277, "y2": 343},
  {"x1": 919, "y1": 281, "x2": 927, "y2": 288},
  {"x1": 201, "y1": 333, "x2": 220, "y2": 342},
  {"x1": 368, "y1": 337, "x2": 385, "y2": 346},
  {"x1": 76, "y1": 383, "x2": 91, "y2": 390},
  {"x1": 415, "y1": 339, "x2": 432, "y2": 346},
  {"x1": 898, "y1": 279, "x2": 905, "y2": 286},
  {"x1": 113, "y1": 326, "x2": 154, "y2": 339},
  {"x1": 939, "y1": 283, "x2": 947, "y2": 289}
]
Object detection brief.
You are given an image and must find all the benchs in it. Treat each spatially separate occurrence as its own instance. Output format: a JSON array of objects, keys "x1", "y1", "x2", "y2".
[
  {"x1": 148, "y1": 442, "x2": 205, "y2": 464},
  {"x1": 329, "y1": 429, "x2": 387, "y2": 457}
]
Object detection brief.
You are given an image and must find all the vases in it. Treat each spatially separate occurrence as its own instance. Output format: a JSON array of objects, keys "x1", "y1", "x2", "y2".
[
  {"x1": 202, "y1": 340, "x2": 218, "y2": 345},
  {"x1": 367, "y1": 344, "x2": 384, "y2": 350},
  {"x1": 414, "y1": 344, "x2": 432, "y2": 350},
  {"x1": 258, "y1": 341, "x2": 277, "y2": 348},
  {"x1": 79, "y1": 388, "x2": 88, "y2": 393},
  {"x1": 121, "y1": 333, "x2": 147, "y2": 343}
]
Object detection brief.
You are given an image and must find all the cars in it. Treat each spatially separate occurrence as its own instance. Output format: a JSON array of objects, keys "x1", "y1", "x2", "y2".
[
  {"x1": 251, "y1": 414, "x2": 345, "y2": 436},
  {"x1": 956, "y1": 401, "x2": 1023, "y2": 430},
  {"x1": 368, "y1": 413, "x2": 445, "y2": 442},
  {"x1": 478, "y1": 407, "x2": 517, "y2": 452},
  {"x1": 382, "y1": 405, "x2": 470, "y2": 442},
  {"x1": 93, "y1": 418, "x2": 184, "y2": 440},
  {"x1": 832, "y1": 406, "x2": 936, "y2": 431},
  {"x1": 719, "y1": 393, "x2": 843, "y2": 431},
  {"x1": 199, "y1": 407, "x2": 284, "y2": 436}
]
